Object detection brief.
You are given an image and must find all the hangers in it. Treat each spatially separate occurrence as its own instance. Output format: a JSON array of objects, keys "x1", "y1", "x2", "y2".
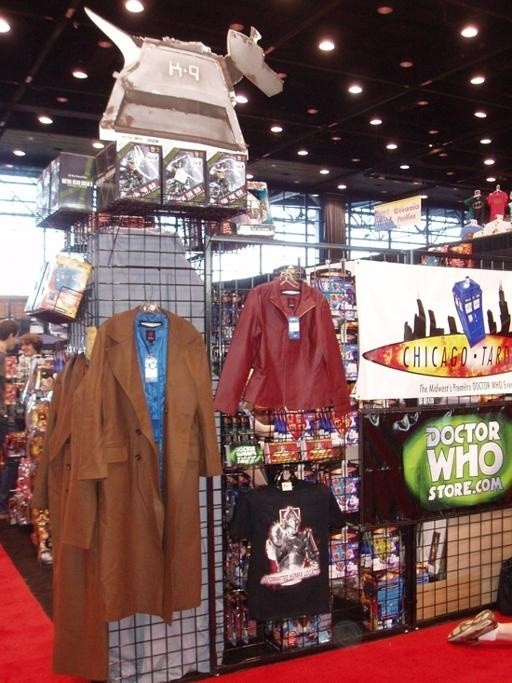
[
  {"x1": 136, "y1": 282, "x2": 166, "y2": 331},
  {"x1": 280, "y1": 263, "x2": 301, "y2": 295},
  {"x1": 272, "y1": 463, "x2": 299, "y2": 485}
]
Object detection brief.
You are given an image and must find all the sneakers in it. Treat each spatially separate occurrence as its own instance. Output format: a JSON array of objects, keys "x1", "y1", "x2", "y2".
[{"x1": 447, "y1": 609, "x2": 499, "y2": 642}]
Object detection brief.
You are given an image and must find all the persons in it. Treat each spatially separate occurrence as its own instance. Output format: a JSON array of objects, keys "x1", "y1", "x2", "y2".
[{"x1": 0, "y1": 320, "x2": 43, "y2": 520}]
